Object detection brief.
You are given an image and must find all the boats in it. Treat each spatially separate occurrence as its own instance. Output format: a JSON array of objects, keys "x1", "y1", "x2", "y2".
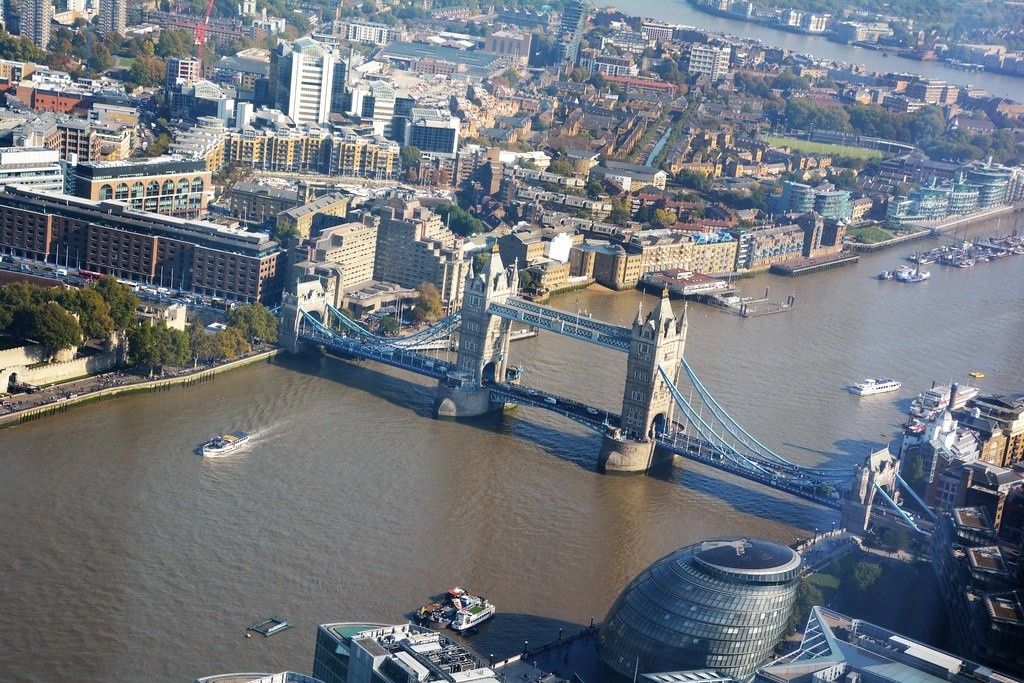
[
  {"x1": 201, "y1": 429, "x2": 250, "y2": 455},
  {"x1": 910, "y1": 381, "x2": 979, "y2": 418},
  {"x1": 908, "y1": 230, "x2": 1024, "y2": 268},
  {"x1": 878, "y1": 265, "x2": 930, "y2": 283},
  {"x1": 450, "y1": 599, "x2": 496, "y2": 632},
  {"x1": 850, "y1": 377, "x2": 901, "y2": 396}
]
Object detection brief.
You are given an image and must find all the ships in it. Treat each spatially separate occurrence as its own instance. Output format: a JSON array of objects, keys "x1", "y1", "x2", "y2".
[{"x1": 415, "y1": 587, "x2": 485, "y2": 629}]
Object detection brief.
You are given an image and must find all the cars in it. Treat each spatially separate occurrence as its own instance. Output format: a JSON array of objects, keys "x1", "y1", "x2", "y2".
[
  {"x1": 528, "y1": 389, "x2": 537, "y2": 396},
  {"x1": 424, "y1": 362, "x2": 434, "y2": 368},
  {"x1": 437, "y1": 366, "x2": 447, "y2": 372},
  {"x1": 587, "y1": 407, "x2": 598, "y2": 415},
  {"x1": 544, "y1": 397, "x2": 556, "y2": 405},
  {"x1": 496, "y1": 382, "x2": 511, "y2": 390}
]
